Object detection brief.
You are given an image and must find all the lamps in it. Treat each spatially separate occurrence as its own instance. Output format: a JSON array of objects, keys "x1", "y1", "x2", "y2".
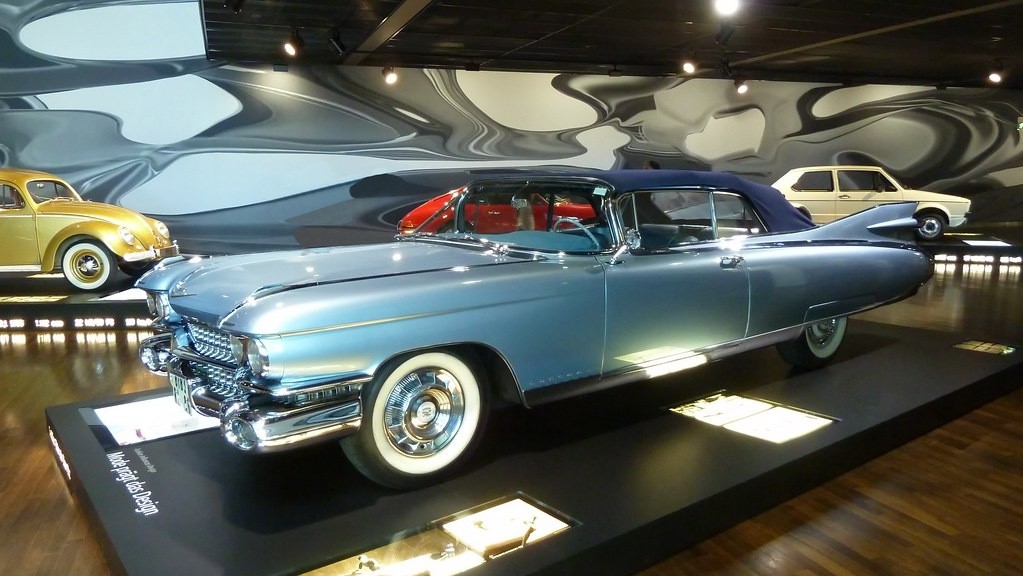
[
  {"x1": 283, "y1": 26, "x2": 304, "y2": 58},
  {"x1": 382, "y1": 57, "x2": 398, "y2": 84},
  {"x1": 683, "y1": 0, "x2": 749, "y2": 94},
  {"x1": 224, "y1": 0, "x2": 246, "y2": 15},
  {"x1": 989, "y1": 58, "x2": 1006, "y2": 83},
  {"x1": 328, "y1": 29, "x2": 349, "y2": 58}
]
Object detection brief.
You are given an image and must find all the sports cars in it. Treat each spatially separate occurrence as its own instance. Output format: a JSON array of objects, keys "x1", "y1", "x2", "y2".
[
  {"x1": 135, "y1": 169, "x2": 936, "y2": 490},
  {"x1": 395, "y1": 182, "x2": 599, "y2": 235},
  {"x1": 765, "y1": 164, "x2": 976, "y2": 241}
]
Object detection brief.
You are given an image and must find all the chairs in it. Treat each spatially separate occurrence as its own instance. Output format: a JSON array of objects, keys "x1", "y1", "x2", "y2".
[{"x1": 13, "y1": 189, "x2": 43, "y2": 207}]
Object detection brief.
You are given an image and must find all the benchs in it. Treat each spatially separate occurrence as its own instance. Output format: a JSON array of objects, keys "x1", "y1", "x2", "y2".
[{"x1": 640, "y1": 224, "x2": 760, "y2": 247}]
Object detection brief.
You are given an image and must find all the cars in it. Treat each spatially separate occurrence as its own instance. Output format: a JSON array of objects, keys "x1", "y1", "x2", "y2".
[{"x1": 0, "y1": 166, "x2": 181, "y2": 298}]
